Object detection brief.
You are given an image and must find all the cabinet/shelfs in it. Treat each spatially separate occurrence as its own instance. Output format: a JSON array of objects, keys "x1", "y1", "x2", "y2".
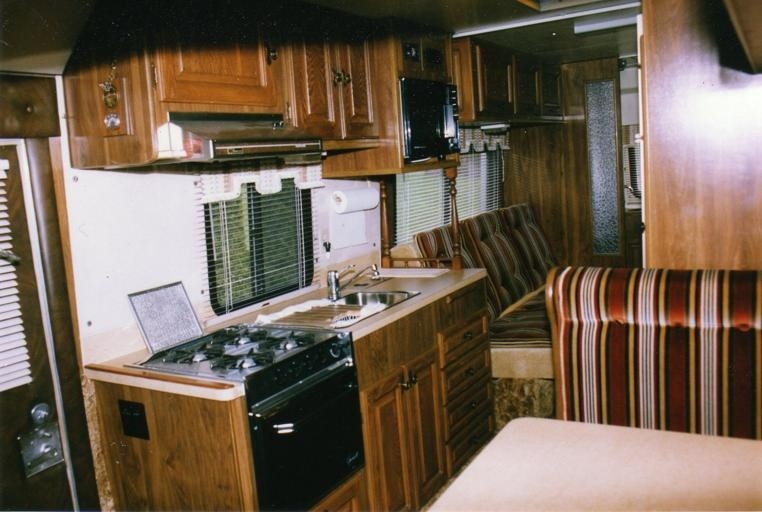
[
  {"x1": 436, "y1": 276, "x2": 496, "y2": 479},
  {"x1": 323, "y1": 20, "x2": 461, "y2": 178},
  {"x1": 352, "y1": 304, "x2": 446, "y2": 512},
  {"x1": 516, "y1": 49, "x2": 540, "y2": 124},
  {"x1": 286, "y1": 12, "x2": 382, "y2": 141},
  {"x1": 455, "y1": 37, "x2": 514, "y2": 125},
  {"x1": 636, "y1": 1, "x2": 762, "y2": 269},
  {"x1": 62, "y1": 1, "x2": 286, "y2": 170},
  {"x1": 83, "y1": 333, "x2": 367, "y2": 512},
  {"x1": 542, "y1": 62, "x2": 563, "y2": 120}
]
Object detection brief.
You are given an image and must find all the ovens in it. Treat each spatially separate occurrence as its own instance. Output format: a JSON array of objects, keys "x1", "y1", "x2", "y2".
[{"x1": 248, "y1": 358, "x2": 368, "y2": 510}]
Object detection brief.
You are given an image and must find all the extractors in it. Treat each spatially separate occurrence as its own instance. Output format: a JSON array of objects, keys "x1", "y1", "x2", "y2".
[{"x1": 105, "y1": 112, "x2": 327, "y2": 171}]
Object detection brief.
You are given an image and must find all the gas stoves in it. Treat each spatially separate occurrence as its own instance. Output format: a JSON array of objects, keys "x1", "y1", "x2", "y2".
[{"x1": 149, "y1": 326, "x2": 351, "y2": 403}]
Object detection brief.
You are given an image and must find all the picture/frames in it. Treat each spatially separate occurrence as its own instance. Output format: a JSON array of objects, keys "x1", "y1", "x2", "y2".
[{"x1": 128, "y1": 280, "x2": 204, "y2": 354}]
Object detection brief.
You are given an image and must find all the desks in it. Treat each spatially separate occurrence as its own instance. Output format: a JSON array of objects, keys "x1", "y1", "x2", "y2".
[{"x1": 424, "y1": 417, "x2": 762, "y2": 512}]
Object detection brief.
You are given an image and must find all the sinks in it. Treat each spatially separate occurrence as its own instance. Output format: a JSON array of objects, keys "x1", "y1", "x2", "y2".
[{"x1": 267, "y1": 290, "x2": 421, "y2": 328}]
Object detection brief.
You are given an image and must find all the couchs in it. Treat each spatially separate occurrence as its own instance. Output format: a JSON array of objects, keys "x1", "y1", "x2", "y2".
[
  {"x1": 414, "y1": 202, "x2": 556, "y2": 434},
  {"x1": 546, "y1": 265, "x2": 761, "y2": 440}
]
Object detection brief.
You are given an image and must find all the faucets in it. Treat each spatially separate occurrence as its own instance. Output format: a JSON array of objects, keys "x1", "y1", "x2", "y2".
[{"x1": 328, "y1": 264, "x2": 377, "y2": 302}]
